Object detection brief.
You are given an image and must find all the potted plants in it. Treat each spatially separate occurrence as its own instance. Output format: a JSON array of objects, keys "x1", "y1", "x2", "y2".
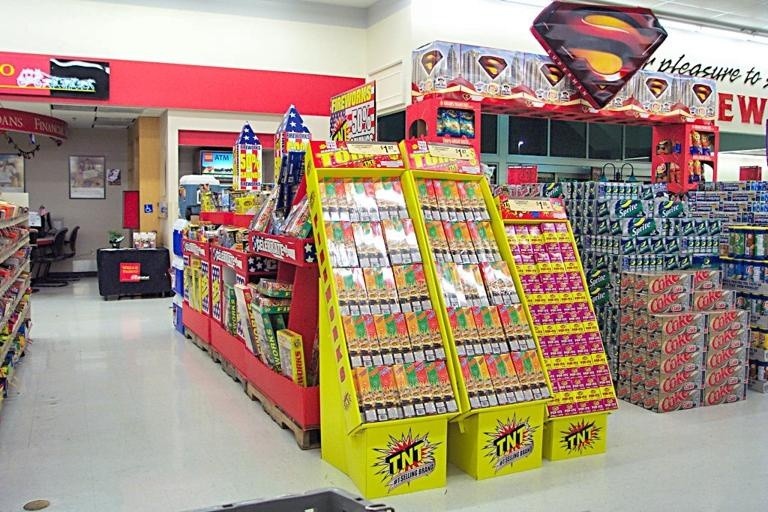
[{"x1": 107, "y1": 230, "x2": 124, "y2": 248}]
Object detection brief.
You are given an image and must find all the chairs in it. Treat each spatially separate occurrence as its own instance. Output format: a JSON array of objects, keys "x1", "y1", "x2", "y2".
[
  {"x1": 43, "y1": 226, "x2": 80, "y2": 282},
  {"x1": 29, "y1": 226, "x2": 68, "y2": 288}
]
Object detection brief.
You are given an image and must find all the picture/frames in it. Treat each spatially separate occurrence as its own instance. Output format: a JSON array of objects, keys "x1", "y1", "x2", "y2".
[
  {"x1": 0, "y1": 153, "x2": 25, "y2": 193},
  {"x1": 68, "y1": 154, "x2": 105, "y2": 200}
]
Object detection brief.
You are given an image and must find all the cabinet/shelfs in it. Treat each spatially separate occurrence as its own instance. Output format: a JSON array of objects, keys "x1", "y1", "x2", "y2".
[{"x1": 0, "y1": 192, "x2": 31, "y2": 407}]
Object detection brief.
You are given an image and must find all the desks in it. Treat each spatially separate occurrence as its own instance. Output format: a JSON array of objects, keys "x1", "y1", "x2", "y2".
[{"x1": 97, "y1": 248, "x2": 171, "y2": 301}]
